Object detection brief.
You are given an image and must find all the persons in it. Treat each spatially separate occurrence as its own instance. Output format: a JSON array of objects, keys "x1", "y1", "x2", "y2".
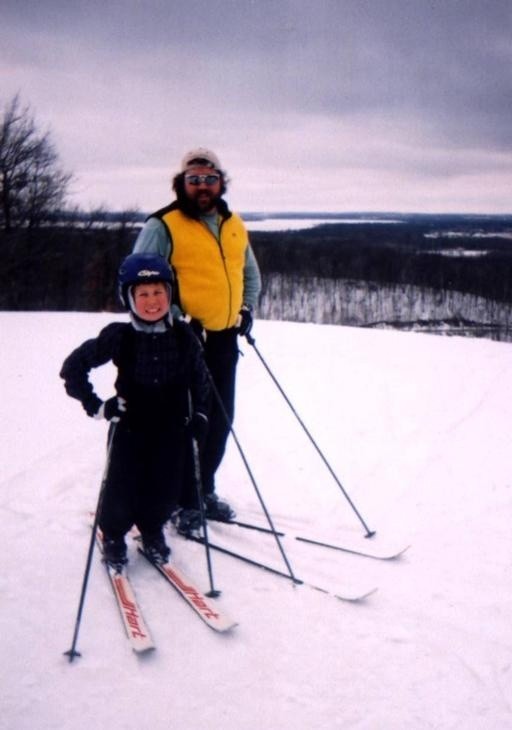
[
  {"x1": 57, "y1": 249, "x2": 218, "y2": 570},
  {"x1": 127, "y1": 148, "x2": 265, "y2": 530}
]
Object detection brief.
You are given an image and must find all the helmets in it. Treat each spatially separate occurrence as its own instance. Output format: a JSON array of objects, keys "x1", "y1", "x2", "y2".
[{"x1": 115, "y1": 251, "x2": 178, "y2": 312}]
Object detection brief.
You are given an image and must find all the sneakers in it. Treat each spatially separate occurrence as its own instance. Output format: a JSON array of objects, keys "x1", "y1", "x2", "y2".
[
  {"x1": 171, "y1": 505, "x2": 207, "y2": 540},
  {"x1": 99, "y1": 530, "x2": 129, "y2": 575},
  {"x1": 138, "y1": 530, "x2": 172, "y2": 565},
  {"x1": 205, "y1": 493, "x2": 235, "y2": 521}
]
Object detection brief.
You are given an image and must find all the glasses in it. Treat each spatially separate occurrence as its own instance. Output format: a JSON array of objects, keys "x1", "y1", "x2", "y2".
[{"x1": 181, "y1": 171, "x2": 221, "y2": 187}]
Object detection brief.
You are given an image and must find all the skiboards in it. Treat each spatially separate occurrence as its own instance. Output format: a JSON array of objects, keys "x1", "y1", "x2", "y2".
[
  {"x1": 164, "y1": 498, "x2": 410, "y2": 602},
  {"x1": 88, "y1": 511, "x2": 239, "y2": 652}
]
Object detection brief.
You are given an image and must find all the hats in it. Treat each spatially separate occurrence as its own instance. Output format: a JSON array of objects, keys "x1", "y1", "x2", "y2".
[{"x1": 179, "y1": 147, "x2": 223, "y2": 174}]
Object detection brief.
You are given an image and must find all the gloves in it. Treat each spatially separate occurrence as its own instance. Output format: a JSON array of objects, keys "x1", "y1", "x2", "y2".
[
  {"x1": 90, "y1": 395, "x2": 126, "y2": 424},
  {"x1": 182, "y1": 412, "x2": 210, "y2": 442},
  {"x1": 232, "y1": 303, "x2": 254, "y2": 338},
  {"x1": 184, "y1": 313, "x2": 209, "y2": 345}
]
